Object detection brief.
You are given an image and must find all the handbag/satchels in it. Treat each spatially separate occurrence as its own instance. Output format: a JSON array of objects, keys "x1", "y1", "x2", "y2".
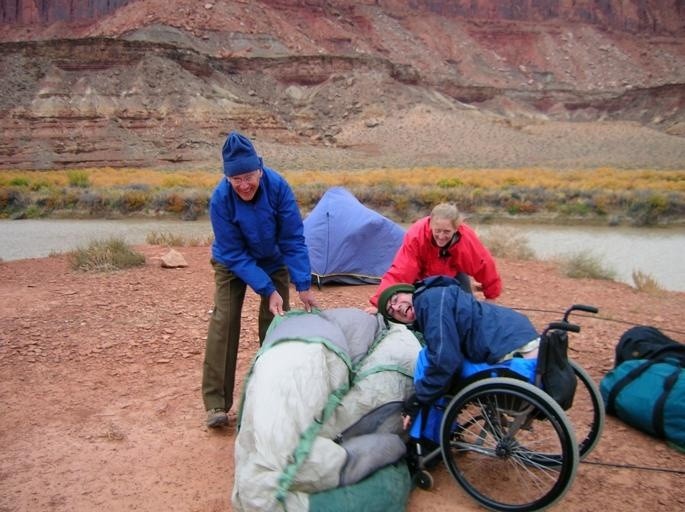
[{"x1": 600, "y1": 326, "x2": 685, "y2": 453}]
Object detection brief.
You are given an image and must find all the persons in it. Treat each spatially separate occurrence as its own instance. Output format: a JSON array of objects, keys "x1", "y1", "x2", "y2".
[
  {"x1": 202, "y1": 132, "x2": 322, "y2": 427},
  {"x1": 377, "y1": 274, "x2": 541, "y2": 468},
  {"x1": 364, "y1": 203, "x2": 504, "y2": 315}
]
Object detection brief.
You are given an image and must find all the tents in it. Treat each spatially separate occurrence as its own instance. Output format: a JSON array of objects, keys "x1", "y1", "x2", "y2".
[{"x1": 303, "y1": 186, "x2": 408, "y2": 290}]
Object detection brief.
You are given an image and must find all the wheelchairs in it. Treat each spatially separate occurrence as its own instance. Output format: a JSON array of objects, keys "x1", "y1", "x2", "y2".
[{"x1": 404, "y1": 304, "x2": 606, "y2": 511}]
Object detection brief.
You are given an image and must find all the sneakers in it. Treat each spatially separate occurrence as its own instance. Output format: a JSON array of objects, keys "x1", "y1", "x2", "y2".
[{"x1": 207, "y1": 409, "x2": 228, "y2": 427}]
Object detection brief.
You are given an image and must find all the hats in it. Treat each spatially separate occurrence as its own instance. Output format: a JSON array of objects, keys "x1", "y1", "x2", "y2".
[
  {"x1": 222, "y1": 133, "x2": 260, "y2": 177},
  {"x1": 378, "y1": 284, "x2": 416, "y2": 325}
]
